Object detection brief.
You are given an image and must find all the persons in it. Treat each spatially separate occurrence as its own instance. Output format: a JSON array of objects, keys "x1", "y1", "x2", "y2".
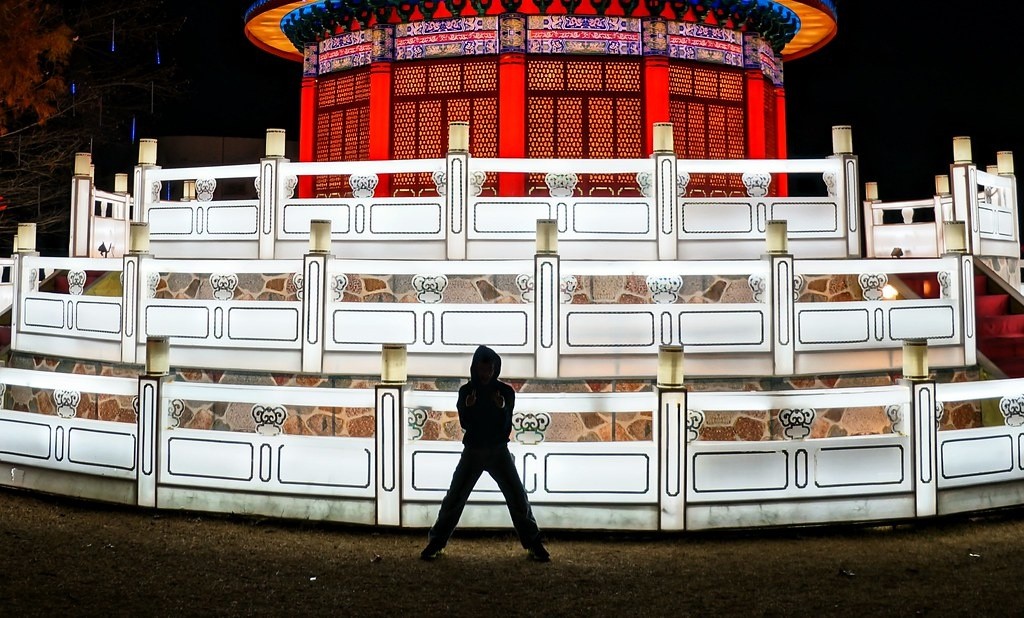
[{"x1": 420, "y1": 345, "x2": 553, "y2": 563}]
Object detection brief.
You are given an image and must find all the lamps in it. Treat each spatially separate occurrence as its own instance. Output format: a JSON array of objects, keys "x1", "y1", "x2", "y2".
[
  {"x1": 137, "y1": 138, "x2": 158, "y2": 166},
  {"x1": 309, "y1": 219, "x2": 332, "y2": 255},
  {"x1": 380, "y1": 343, "x2": 407, "y2": 382},
  {"x1": 942, "y1": 221, "x2": 967, "y2": 255},
  {"x1": 764, "y1": 220, "x2": 789, "y2": 255},
  {"x1": 128, "y1": 222, "x2": 150, "y2": 255},
  {"x1": 12, "y1": 222, "x2": 36, "y2": 254},
  {"x1": 656, "y1": 345, "x2": 684, "y2": 389},
  {"x1": 536, "y1": 219, "x2": 558, "y2": 253},
  {"x1": 73, "y1": 152, "x2": 95, "y2": 188},
  {"x1": 934, "y1": 136, "x2": 1015, "y2": 195},
  {"x1": 447, "y1": 121, "x2": 469, "y2": 153},
  {"x1": 145, "y1": 336, "x2": 170, "y2": 376},
  {"x1": 114, "y1": 173, "x2": 128, "y2": 194},
  {"x1": 265, "y1": 128, "x2": 286, "y2": 159},
  {"x1": 652, "y1": 122, "x2": 674, "y2": 154},
  {"x1": 183, "y1": 180, "x2": 196, "y2": 200},
  {"x1": 865, "y1": 181, "x2": 878, "y2": 201},
  {"x1": 902, "y1": 337, "x2": 929, "y2": 380},
  {"x1": 831, "y1": 125, "x2": 853, "y2": 155}
]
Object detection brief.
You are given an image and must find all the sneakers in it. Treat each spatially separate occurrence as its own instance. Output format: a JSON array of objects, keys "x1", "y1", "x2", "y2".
[
  {"x1": 421, "y1": 538, "x2": 446, "y2": 558},
  {"x1": 527, "y1": 544, "x2": 550, "y2": 561}
]
